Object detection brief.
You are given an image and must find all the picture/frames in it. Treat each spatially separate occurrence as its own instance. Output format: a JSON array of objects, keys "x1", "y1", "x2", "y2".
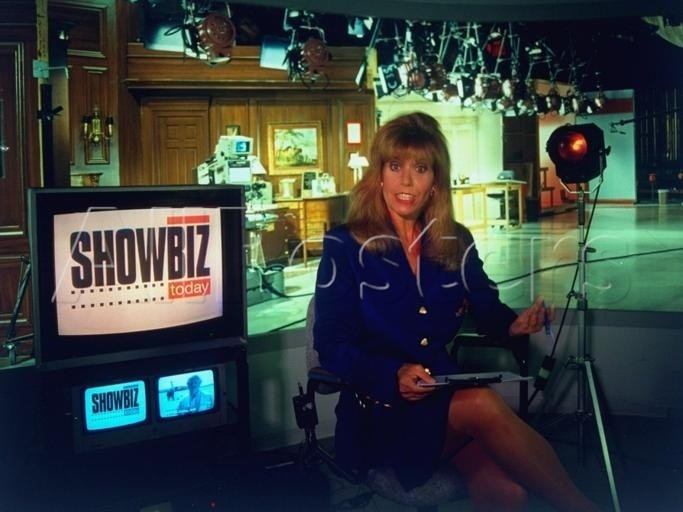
[
  {"x1": 344, "y1": 119, "x2": 364, "y2": 146},
  {"x1": 266, "y1": 120, "x2": 325, "y2": 175}
]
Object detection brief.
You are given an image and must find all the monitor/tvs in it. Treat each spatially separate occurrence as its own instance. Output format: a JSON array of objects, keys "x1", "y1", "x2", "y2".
[
  {"x1": 26, "y1": 185, "x2": 250, "y2": 373},
  {"x1": 219, "y1": 135, "x2": 254, "y2": 156},
  {"x1": 63, "y1": 361, "x2": 231, "y2": 456}
]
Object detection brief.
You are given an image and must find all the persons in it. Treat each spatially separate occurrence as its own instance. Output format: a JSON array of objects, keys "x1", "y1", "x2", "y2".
[
  {"x1": 313, "y1": 113, "x2": 602, "y2": 510},
  {"x1": 176, "y1": 376, "x2": 213, "y2": 415}
]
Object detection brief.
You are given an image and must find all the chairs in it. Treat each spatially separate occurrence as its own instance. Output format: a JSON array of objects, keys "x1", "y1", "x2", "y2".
[
  {"x1": 294, "y1": 293, "x2": 531, "y2": 512},
  {"x1": 505, "y1": 163, "x2": 555, "y2": 221}
]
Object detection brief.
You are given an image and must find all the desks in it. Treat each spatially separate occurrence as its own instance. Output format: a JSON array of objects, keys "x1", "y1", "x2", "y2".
[{"x1": 443, "y1": 179, "x2": 528, "y2": 232}]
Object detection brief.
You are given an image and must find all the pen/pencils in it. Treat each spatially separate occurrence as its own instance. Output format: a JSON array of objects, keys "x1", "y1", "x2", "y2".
[{"x1": 542, "y1": 299, "x2": 551, "y2": 337}]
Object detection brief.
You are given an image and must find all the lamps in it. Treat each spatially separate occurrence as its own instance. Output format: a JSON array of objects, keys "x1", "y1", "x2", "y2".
[
  {"x1": 281, "y1": 10, "x2": 332, "y2": 92},
  {"x1": 347, "y1": 155, "x2": 370, "y2": 186},
  {"x1": 164, "y1": 2, "x2": 236, "y2": 68}
]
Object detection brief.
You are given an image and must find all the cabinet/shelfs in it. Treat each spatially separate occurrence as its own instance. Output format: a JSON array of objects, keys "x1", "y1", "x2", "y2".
[
  {"x1": 0, "y1": 2, "x2": 42, "y2": 352},
  {"x1": 302, "y1": 194, "x2": 346, "y2": 260},
  {"x1": 252, "y1": 94, "x2": 334, "y2": 194},
  {"x1": 333, "y1": 92, "x2": 378, "y2": 222},
  {"x1": 210, "y1": 96, "x2": 251, "y2": 156},
  {"x1": 43, "y1": 2, "x2": 124, "y2": 188},
  {"x1": 141, "y1": 96, "x2": 209, "y2": 184},
  {"x1": 272, "y1": 194, "x2": 303, "y2": 243}
]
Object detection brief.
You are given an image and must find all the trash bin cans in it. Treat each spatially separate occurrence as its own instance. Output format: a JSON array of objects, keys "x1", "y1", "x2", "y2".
[{"x1": 658, "y1": 190, "x2": 670, "y2": 204}]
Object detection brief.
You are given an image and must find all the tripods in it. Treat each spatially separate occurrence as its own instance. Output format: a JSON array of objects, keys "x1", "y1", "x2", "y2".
[{"x1": 525, "y1": 193, "x2": 628, "y2": 511}]
